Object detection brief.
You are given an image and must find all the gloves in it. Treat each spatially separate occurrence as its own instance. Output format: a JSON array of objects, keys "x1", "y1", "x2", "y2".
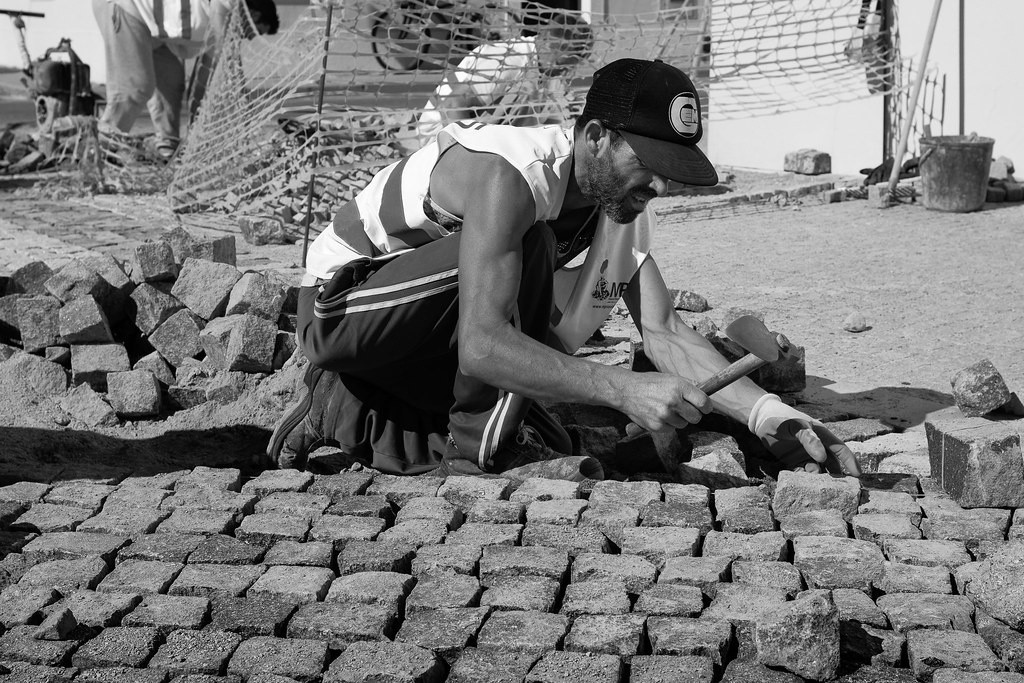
[{"x1": 748, "y1": 392, "x2": 862, "y2": 479}]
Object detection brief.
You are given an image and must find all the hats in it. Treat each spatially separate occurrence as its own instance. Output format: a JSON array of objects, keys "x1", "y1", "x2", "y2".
[{"x1": 583, "y1": 59, "x2": 719, "y2": 187}]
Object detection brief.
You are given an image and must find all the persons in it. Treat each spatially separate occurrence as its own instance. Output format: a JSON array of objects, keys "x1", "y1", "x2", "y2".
[
  {"x1": 265, "y1": 59, "x2": 861, "y2": 480},
  {"x1": 92, "y1": 0, "x2": 280, "y2": 168},
  {"x1": 416, "y1": 14, "x2": 595, "y2": 147}
]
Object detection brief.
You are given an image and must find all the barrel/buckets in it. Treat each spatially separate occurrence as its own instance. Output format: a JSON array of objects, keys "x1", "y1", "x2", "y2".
[{"x1": 919, "y1": 134, "x2": 996, "y2": 213}]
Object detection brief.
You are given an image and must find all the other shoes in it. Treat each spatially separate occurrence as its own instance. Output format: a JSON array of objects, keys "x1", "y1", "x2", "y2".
[{"x1": 155, "y1": 139, "x2": 175, "y2": 160}]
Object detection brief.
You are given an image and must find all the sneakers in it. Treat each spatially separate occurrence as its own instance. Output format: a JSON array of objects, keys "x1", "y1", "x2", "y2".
[
  {"x1": 266, "y1": 359, "x2": 338, "y2": 468},
  {"x1": 439, "y1": 421, "x2": 569, "y2": 478}
]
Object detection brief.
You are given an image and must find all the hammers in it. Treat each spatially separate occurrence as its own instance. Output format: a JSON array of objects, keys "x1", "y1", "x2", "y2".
[{"x1": 626, "y1": 315, "x2": 801, "y2": 440}]
